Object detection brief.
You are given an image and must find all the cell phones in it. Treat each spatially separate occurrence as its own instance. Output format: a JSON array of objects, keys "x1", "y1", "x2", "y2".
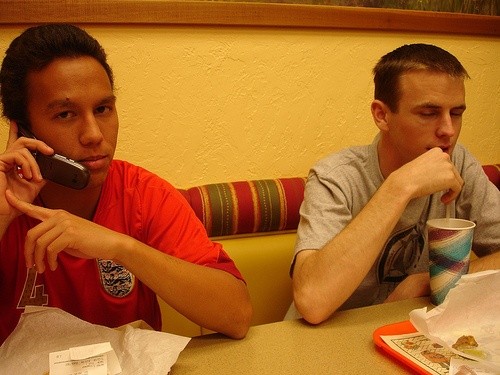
[{"x1": 13, "y1": 121, "x2": 90, "y2": 190}]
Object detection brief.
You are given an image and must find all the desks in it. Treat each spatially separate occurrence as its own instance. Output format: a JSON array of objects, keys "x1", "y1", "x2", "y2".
[{"x1": 167, "y1": 296, "x2": 500, "y2": 375}]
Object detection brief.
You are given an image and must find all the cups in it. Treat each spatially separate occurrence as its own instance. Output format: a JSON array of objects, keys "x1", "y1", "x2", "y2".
[{"x1": 427, "y1": 218, "x2": 475, "y2": 306}]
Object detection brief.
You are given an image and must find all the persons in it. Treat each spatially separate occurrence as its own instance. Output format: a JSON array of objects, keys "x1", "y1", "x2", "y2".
[
  {"x1": 283, "y1": 43, "x2": 500, "y2": 325},
  {"x1": 0, "y1": 23, "x2": 253, "y2": 347}
]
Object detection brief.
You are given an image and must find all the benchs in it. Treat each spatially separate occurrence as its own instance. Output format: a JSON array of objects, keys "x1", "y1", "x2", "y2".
[{"x1": 157, "y1": 163, "x2": 500, "y2": 339}]
